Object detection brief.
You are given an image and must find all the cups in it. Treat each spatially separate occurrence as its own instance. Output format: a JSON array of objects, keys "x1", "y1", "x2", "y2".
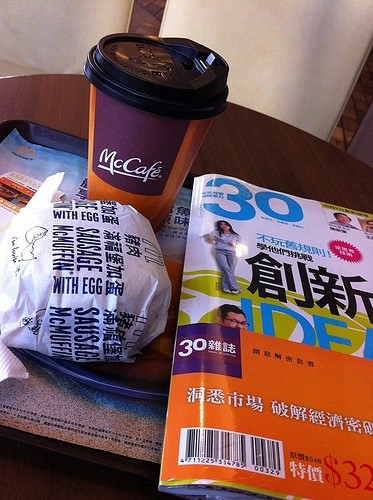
[{"x1": 82, "y1": 33, "x2": 230, "y2": 234}]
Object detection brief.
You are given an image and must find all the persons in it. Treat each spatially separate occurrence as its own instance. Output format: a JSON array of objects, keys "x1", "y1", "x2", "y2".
[
  {"x1": 329, "y1": 211, "x2": 359, "y2": 229},
  {"x1": 207, "y1": 219, "x2": 243, "y2": 294},
  {"x1": 217, "y1": 304, "x2": 248, "y2": 331}
]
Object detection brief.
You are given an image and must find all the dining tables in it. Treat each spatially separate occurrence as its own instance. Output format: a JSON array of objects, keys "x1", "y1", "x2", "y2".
[{"x1": 1, "y1": 73, "x2": 373, "y2": 500}]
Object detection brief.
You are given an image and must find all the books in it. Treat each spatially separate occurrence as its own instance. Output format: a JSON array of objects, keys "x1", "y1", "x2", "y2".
[{"x1": 156, "y1": 171, "x2": 373, "y2": 500}]
